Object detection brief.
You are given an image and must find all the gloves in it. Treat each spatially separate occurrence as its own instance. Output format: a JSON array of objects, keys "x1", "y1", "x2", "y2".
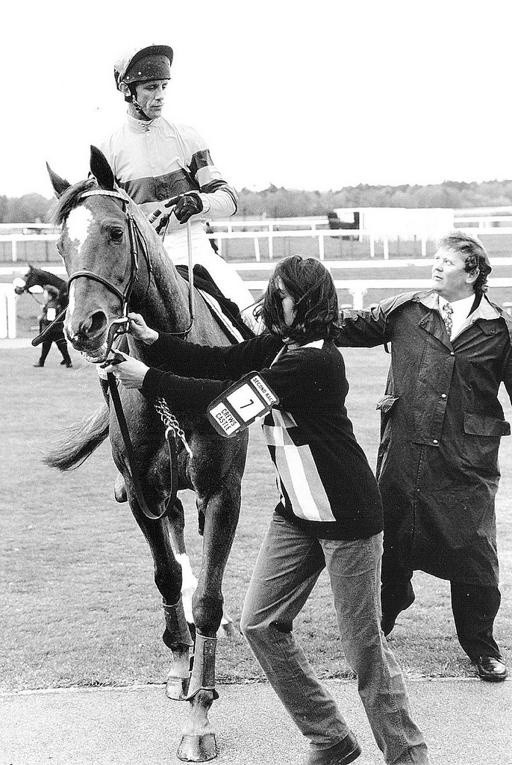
[{"x1": 165, "y1": 193, "x2": 203, "y2": 224}]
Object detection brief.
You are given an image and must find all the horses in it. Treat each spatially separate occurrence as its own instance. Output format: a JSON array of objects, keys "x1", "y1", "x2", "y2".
[
  {"x1": 40, "y1": 144, "x2": 251, "y2": 764},
  {"x1": 10, "y1": 258, "x2": 66, "y2": 314}
]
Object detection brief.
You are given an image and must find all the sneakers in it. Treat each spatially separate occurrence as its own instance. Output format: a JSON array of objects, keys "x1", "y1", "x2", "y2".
[{"x1": 307, "y1": 730, "x2": 361, "y2": 765}]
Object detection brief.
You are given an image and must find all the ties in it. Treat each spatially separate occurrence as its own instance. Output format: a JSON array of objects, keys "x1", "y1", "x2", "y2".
[{"x1": 442, "y1": 303, "x2": 453, "y2": 342}]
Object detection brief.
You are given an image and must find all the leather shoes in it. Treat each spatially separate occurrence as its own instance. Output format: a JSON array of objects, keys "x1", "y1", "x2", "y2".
[
  {"x1": 475, "y1": 654, "x2": 507, "y2": 682},
  {"x1": 381, "y1": 591, "x2": 415, "y2": 637}
]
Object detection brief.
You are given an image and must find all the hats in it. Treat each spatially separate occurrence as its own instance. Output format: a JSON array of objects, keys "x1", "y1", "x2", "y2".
[{"x1": 114, "y1": 42, "x2": 173, "y2": 90}]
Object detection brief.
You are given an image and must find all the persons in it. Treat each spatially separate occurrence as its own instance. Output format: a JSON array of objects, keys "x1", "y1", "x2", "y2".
[
  {"x1": 33, "y1": 284, "x2": 74, "y2": 369},
  {"x1": 89, "y1": 35, "x2": 265, "y2": 503},
  {"x1": 276, "y1": 231, "x2": 512, "y2": 681},
  {"x1": 107, "y1": 254, "x2": 428, "y2": 763}
]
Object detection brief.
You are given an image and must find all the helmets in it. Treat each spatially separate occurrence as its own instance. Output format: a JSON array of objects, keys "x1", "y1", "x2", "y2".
[{"x1": 122, "y1": 54, "x2": 171, "y2": 84}]
